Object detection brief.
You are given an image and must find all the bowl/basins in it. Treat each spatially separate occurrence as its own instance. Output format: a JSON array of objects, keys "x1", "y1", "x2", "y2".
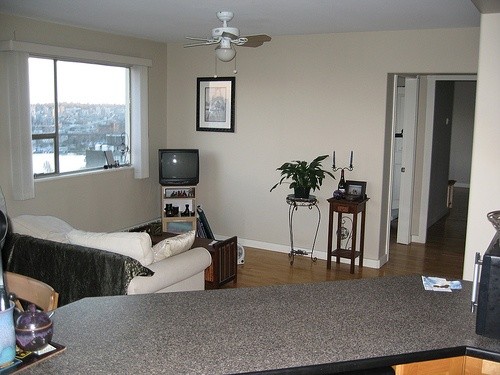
[
  {"x1": 487, "y1": 210, "x2": 500, "y2": 239},
  {"x1": 15, "y1": 303, "x2": 53, "y2": 351}
]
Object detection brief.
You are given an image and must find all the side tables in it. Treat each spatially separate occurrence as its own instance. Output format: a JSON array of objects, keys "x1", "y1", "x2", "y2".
[{"x1": 327, "y1": 197, "x2": 370, "y2": 274}]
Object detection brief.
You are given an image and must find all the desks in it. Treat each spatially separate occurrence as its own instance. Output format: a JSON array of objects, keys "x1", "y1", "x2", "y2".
[{"x1": 1, "y1": 273, "x2": 500, "y2": 375}]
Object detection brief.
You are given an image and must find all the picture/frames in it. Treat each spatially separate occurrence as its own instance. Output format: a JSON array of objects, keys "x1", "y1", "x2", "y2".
[
  {"x1": 344, "y1": 180, "x2": 367, "y2": 200},
  {"x1": 196, "y1": 76, "x2": 236, "y2": 133}
]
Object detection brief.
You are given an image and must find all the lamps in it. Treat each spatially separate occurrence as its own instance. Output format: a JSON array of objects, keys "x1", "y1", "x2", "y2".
[{"x1": 216, "y1": 38, "x2": 236, "y2": 62}]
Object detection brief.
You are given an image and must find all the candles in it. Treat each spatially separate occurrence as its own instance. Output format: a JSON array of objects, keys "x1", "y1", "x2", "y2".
[
  {"x1": 333, "y1": 150, "x2": 335, "y2": 165},
  {"x1": 350, "y1": 151, "x2": 353, "y2": 167}
]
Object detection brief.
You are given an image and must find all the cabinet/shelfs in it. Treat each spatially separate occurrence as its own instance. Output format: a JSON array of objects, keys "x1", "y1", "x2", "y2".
[{"x1": 161, "y1": 186, "x2": 196, "y2": 234}]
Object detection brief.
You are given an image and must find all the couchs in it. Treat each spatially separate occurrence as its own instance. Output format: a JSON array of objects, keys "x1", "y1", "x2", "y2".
[{"x1": 0, "y1": 215, "x2": 213, "y2": 310}]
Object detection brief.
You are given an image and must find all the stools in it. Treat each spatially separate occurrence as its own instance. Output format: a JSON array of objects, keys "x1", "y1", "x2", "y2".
[{"x1": 447, "y1": 179, "x2": 457, "y2": 208}]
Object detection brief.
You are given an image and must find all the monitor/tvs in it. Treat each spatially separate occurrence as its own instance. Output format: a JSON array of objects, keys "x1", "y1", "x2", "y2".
[{"x1": 158, "y1": 148, "x2": 199, "y2": 186}]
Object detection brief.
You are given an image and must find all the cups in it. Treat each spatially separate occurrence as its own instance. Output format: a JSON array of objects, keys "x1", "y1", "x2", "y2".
[{"x1": 0, "y1": 300, "x2": 16, "y2": 367}]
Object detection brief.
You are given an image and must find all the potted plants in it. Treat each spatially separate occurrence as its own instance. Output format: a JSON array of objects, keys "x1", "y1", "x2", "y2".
[{"x1": 269, "y1": 155, "x2": 335, "y2": 198}]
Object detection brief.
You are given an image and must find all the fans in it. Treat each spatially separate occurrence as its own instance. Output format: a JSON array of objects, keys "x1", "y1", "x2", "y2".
[{"x1": 183, "y1": 11, "x2": 271, "y2": 48}]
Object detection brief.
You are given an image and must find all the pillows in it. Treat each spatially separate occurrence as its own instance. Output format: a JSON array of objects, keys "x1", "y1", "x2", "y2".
[{"x1": 153, "y1": 230, "x2": 196, "y2": 263}]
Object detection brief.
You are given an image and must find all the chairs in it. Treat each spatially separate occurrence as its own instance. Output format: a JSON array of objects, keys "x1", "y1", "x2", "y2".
[{"x1": 5, "y1": 271, "x2": 59, "y2": 313}]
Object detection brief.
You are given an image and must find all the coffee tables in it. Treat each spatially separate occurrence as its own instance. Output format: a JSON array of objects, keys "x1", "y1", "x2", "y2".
[{"x1": 151, "y1": 232, "x2": 237, "y2": 290}]
